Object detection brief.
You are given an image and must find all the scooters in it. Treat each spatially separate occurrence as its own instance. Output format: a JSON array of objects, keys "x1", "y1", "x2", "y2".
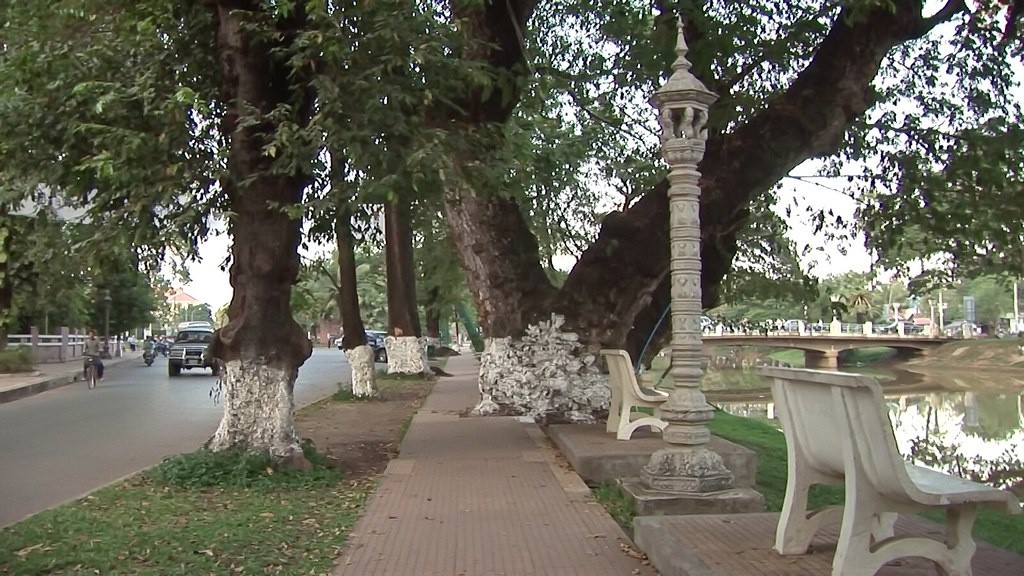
[{"x1": 143, "y1": 343, "x2": 156, "y2": 366}]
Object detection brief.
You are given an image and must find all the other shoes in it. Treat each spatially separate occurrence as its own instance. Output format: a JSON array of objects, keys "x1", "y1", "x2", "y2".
[
  {"x1": 80, "y1": 377, "x2": 86, "y2": 381},
  {"x1": 98, "y1": 378, "x2": 104, "y2": 381}
]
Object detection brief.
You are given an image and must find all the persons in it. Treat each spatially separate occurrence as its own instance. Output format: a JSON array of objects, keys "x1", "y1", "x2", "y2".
[
  {"x1": 81, "y1": 330, "x2": 105, "y2": 381},
  {"x1": 143, "y1": 334, "x2": 170, "y2": 358},
  {"x1": 326, "y1": 331, "x2": 332, "y2": 348},
  {"x1": 127, "y1": 334, "x2": 138, "y2": 352}
]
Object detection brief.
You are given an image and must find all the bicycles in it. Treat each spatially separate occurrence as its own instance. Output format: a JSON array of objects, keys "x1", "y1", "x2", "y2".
[{"x1": 83, "y1": 352, "x2": 101, "y2": 390}]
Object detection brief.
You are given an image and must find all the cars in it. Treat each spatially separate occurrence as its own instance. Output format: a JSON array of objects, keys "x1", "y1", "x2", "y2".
[
  {"x1": 327, "y1": 330, "x2": 388, "y2": 362},
  {"x1": 881, "y1": 321, "x2": 924, "y2": 333},
  {"x1": 700, "y1": 315, "x2": 714, "y2": 334},
  {"x1": 784, "y1": 319, "x2": 809, "y2": 332}
]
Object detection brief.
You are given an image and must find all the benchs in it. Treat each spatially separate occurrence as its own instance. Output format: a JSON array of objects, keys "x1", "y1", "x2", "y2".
[
  {"x1": 599, "y1": 350, "x2": 667, "y2": 441},
  {"x1": 756, "y1": 363, "x2": 1024, "y2": 576}
]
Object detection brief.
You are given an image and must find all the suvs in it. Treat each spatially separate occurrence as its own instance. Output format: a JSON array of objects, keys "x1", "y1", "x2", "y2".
[{"x1": 167, "y1": 320, "x2": 223, "y2": 377}]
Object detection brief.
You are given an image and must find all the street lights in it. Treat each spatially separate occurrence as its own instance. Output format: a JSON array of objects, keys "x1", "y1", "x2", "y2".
[{"x1": 646, "y1": 15, "x2": 734, "y2": 491}]
